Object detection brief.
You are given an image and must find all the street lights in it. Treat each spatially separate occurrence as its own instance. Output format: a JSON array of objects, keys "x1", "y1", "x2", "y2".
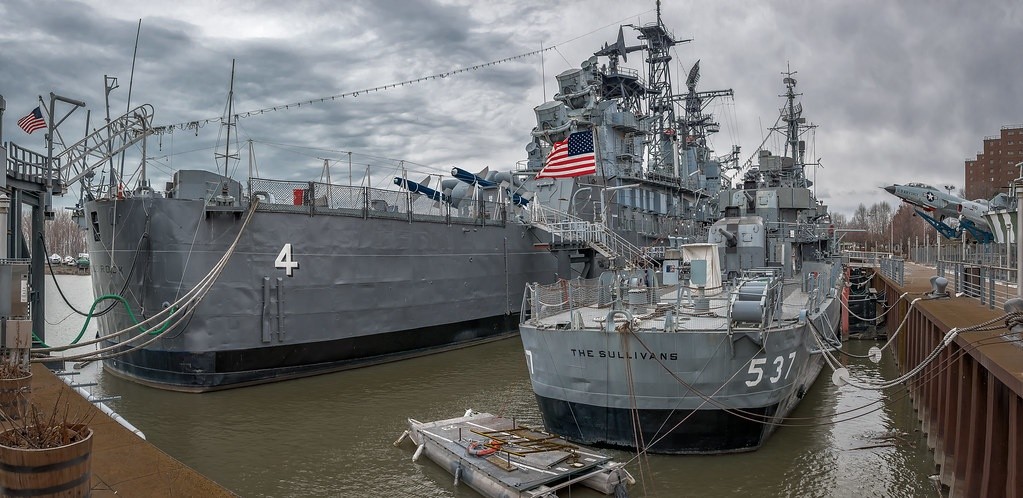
[{"x1": 945, "y1": 184, "x2": 955, "y2": 194}]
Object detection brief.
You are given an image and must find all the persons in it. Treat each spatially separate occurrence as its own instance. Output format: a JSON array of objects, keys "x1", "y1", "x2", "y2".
[
  {"x1": 58, "y1": 258, "x2": 61, "y2": 265},
  {"x1": 792, "y1": 247, "x2": 797, "y2": 275}
]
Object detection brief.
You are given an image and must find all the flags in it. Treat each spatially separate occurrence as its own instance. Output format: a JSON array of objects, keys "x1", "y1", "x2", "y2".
[
  {"x1": 534, "y1": 130, "x2": 597, "y2": 181},
  {"x1": 17, "y1": 107, "x2": 48, "y2": 134},
  {"x1": 663, "y1": 129, "x2": 676, "y2": 134}
]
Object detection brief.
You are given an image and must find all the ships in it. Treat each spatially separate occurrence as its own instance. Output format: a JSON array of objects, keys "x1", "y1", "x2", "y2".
[{"x1": 70, "y1": 18, "x2": 865, "y2": 456}]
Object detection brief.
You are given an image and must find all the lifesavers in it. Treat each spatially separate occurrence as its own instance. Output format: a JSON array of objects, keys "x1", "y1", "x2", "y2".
[
  {"x1": 829, "y1": 224, "x2": 834, "y2": 234},
  {"x1": 468, "y1": 439, "x2": 499, "y2": 455}
]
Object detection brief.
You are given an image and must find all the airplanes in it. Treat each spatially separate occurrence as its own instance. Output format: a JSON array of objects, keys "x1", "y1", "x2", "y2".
[{"x1": 879, "y1": 179, "x2": 1011, "y2": 245}]
